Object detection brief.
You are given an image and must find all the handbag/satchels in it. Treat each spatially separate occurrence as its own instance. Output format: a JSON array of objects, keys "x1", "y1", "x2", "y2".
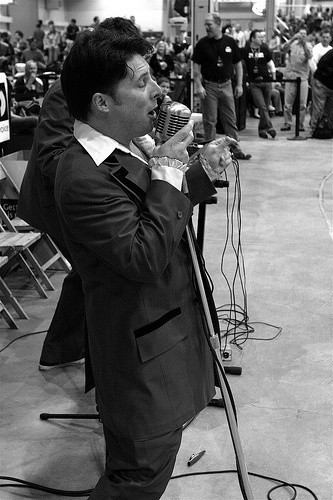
[{"x1": 312, "y1": 114, "x2": 333, "y2": 139}]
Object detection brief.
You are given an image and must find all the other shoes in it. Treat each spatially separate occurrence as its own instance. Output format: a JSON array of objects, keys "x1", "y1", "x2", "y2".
[
  {"x1": 258, "y1": 130, "x2": 268, "y2": 139},
  {"x1": 280, "y1": 124, "x2": 291, "y2": 131},
  {"x1": 234, "y1": 150, "x2": 252, "y2": 161},
  {"x1": 267, "y1": 128, "x2": 277, "y2": 139},
  {"x1": 297, "y1": 126, "x2": 305, "y2": 131},
  {"x1": 38, "y1": 357, "x2": 86, "y2": 371}
]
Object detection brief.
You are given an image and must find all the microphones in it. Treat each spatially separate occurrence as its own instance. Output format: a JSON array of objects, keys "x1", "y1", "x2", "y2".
[{"x1": 155, "y1": 101, "x2": 191, "y2": 147}]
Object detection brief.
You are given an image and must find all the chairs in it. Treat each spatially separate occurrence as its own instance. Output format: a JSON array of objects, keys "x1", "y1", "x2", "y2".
[{"x1": 0, "y1": 161, "x2": 72, "y2": 330}]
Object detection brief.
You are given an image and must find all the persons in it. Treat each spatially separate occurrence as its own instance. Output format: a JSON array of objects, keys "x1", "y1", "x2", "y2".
[
  {"x1": 0, "y1": 6, "x2": 333, "y2": 370},
  {"x1": 56, "y1": 27, "x2": 239, "y2": 500}
]
